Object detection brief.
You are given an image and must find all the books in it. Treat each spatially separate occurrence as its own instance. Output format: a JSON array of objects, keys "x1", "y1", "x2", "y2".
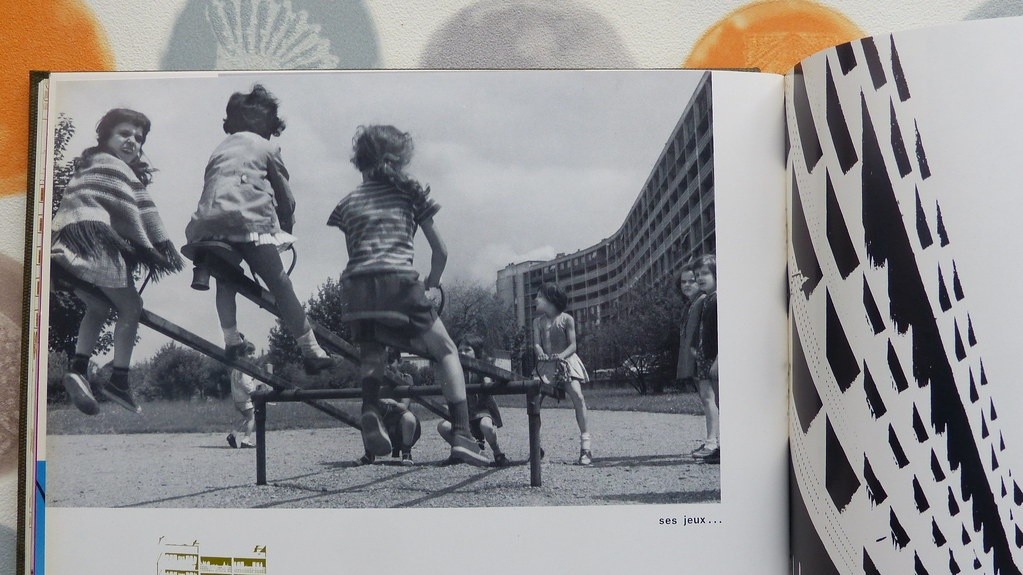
[{"x1": 22, "y1": 18, "x2": 1023, "y2": 575}]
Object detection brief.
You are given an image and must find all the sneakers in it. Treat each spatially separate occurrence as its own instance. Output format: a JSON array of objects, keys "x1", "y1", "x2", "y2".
[{"x1": 692, "y1": 444, "x2": 718, "y2": 457}]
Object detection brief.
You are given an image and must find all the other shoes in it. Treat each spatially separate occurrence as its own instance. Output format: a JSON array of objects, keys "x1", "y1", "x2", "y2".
[
  {"x1": 303, "y1": 345, "x2": 335, "y2": 375},
  {"x1": 494, "y1": 453, "x2": 509, "y2": 466},
  {"x1": 579, "y1": 448, "x2": 592, "y2": 465},
  {"x1": 101, "y1": 380, "x2": 143, "y2": 414},
  {"x1": 227, "y1": 434, "x2": 238, "y2": 448},
  {"x1": 63, "y1": 372, "x2": 99, "y2": 415},
  {"x1": 225, "y1": 333, "x2": 255, "y2": 360},
  {"x1": 402, "y1": 452, "x2": 415, "y2": 466},
  {"x1": 527, "y1": 449, "x2": 545, "y2": 465},
  {"x1": 704, "y1": 449, "x2": 720, "y2": 463},
  {"x1": 241, "y1": 442, "x2": 255, "y2": 448},
  {"x1": 453, "y1": 434, "x2": 491, "y2": 466},
  {"x1": 361, "y1": 412, "x2": 392, "y2": 456}
]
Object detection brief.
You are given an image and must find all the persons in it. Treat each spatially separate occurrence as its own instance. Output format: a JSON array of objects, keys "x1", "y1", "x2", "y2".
[
  {"x1": 350, "y1": 342, "x2": 421, "y2": 465},
  {"x1": 531, "y1": 284, "x2": 593, "y2": 465},
  {"x1": 674, "y1": 255, "x2": 721, "y2": 464},
  {"x1": 226, "y1": 341, "x2": 268, "y2": 448},
  {"x1": 52, "y1": 107, "x2": 159, "y2": 418},
  {"x1": 436, "y1": 334, "x2": 511, "y2": 467},
  {"x1": 325, "y1": 126, "x2": 497, "y2": 466},
  {"x1": 183, "y1": 83, "x2": 344, "y2": 378}
]
